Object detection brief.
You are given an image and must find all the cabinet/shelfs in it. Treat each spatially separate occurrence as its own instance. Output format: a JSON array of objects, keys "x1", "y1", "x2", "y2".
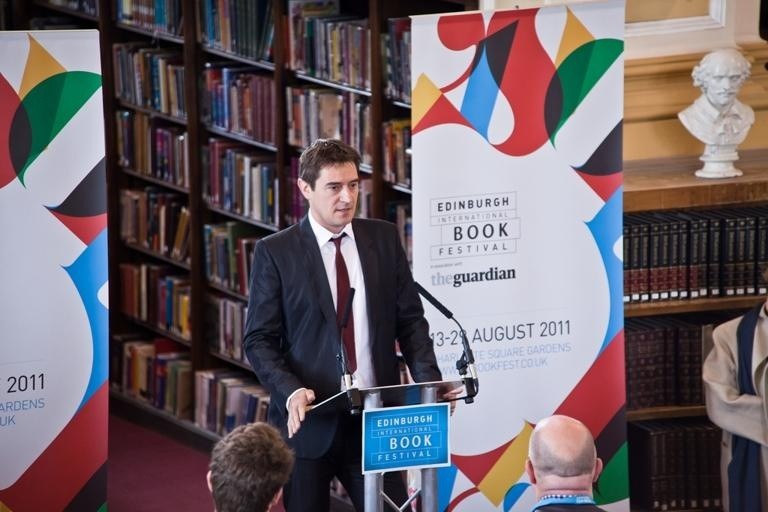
[
  {"x1": 616, "y1": 147, "x2": 767, "y2": 512},
  {"x1": 27, "y1": 0, "x2": 478, "y2": 512}
]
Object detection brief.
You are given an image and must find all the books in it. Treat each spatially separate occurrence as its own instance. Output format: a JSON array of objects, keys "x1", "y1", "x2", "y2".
[
  {"x1": 331, "y1": 478, "x2": 352, "y2": 505},
  {"x1": 29, "y1": 0, "x2": 96, "y2": 30},
  {"x1": 112, "y1": 0, "x2": 421, "y2": 440},
  {"x1": 626, "y1": 420, "x2": 723, "y2": 511},
  {"x1": 625, "y1": 314, "x2": 736, "y2": 409},
  {"x1": 623, "y1": 206, "x2": 768, "y2": 304}
]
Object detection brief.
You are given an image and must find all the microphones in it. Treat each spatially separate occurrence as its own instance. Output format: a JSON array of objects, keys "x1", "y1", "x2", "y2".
[
  {"x1": 414, "y1": 281, "x2": 478, "y2": 379},
  {"x1": 339, "y1": 288, "x2": 355, "y2": 392}
]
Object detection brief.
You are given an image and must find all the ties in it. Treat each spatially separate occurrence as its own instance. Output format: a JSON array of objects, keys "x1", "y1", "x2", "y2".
[{"x1": 329, "y1": 233, "x2": 356, "y2": 374}]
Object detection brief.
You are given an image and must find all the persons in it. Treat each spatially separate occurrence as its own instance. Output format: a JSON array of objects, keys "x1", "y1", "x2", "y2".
[
  {"x1": 243, "y1": 139, "x2": 463, "y2": 511},
  {"x1": 206, "y1": 421, "x2": 295, "y2": 512},
  {"x1": 523, "y1": 416, "x2": 608, "y2": 510},
  {"x1": 675, "y1": 48, "x2": 759, "y2": 179},
  {"x1": 701, "y1": 267, "x2": 768, "y2": 512}
]
